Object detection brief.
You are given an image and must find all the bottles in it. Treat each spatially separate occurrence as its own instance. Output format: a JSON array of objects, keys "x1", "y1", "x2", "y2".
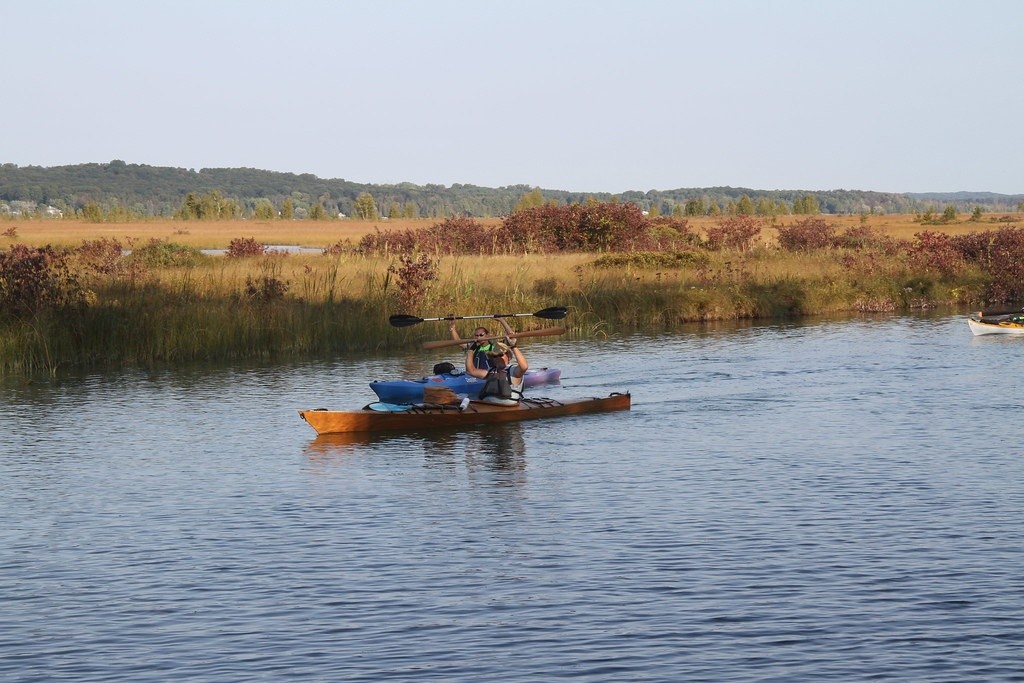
[{"x1": 460, "y1": 397, "x2": 470, "y2": 411}]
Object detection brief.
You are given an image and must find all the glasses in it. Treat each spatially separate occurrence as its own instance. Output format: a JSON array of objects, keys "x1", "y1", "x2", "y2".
[
  {"x1": 474, "y1": 332, "x2": 486, "y2": 337},
  {"x1": 491, "y1": 354, "x2": 506, "y2": 358}
]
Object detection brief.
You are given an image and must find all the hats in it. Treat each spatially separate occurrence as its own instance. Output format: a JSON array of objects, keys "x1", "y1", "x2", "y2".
[{"x1": 486, "y1": 341, "x2": 513, "y2": 361}]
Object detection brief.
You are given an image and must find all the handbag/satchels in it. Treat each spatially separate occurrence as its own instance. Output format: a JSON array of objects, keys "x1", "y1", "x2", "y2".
[{"x1": 424, "y1": 386, "x2": 457, "y2": 404}]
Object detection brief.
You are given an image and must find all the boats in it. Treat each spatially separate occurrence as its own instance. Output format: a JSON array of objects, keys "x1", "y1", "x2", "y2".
[
  {"x1": 968, "y1": 315, "x2": 1024, "y2": 338},
  {"x1": 295, "y1": 391, "x2": 636, "y2": 438},
  {"x1": 367, "y1": 366, "x2": 562, "y2": 401}
]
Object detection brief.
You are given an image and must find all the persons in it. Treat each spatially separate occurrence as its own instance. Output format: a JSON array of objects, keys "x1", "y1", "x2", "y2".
[
  {"x1": 466, "y1": 331, "x2": 528, "y2": 404},
  {"x1": 447, "y1": 312, "x2": 518, "y2": 370}
]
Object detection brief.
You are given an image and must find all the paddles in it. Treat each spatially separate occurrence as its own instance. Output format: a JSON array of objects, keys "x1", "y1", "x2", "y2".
[
  {"x1": 421, "y1": 326, "x2": 565, "y2": 351},
  {"x1": 978, "y1": 309, "x2": 1024, "y2": 318},
  {"x1": 388, "y1": 306, "x2": 568, "y2": 327}
]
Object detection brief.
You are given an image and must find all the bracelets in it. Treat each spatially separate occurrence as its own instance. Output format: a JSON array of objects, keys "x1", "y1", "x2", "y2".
[
  {"x1": 449, "y1": 327, "x2": 456, "y2": 331},
  {"x1": 510, "y1": 346, "x2": 516, "y2": 350}
]
